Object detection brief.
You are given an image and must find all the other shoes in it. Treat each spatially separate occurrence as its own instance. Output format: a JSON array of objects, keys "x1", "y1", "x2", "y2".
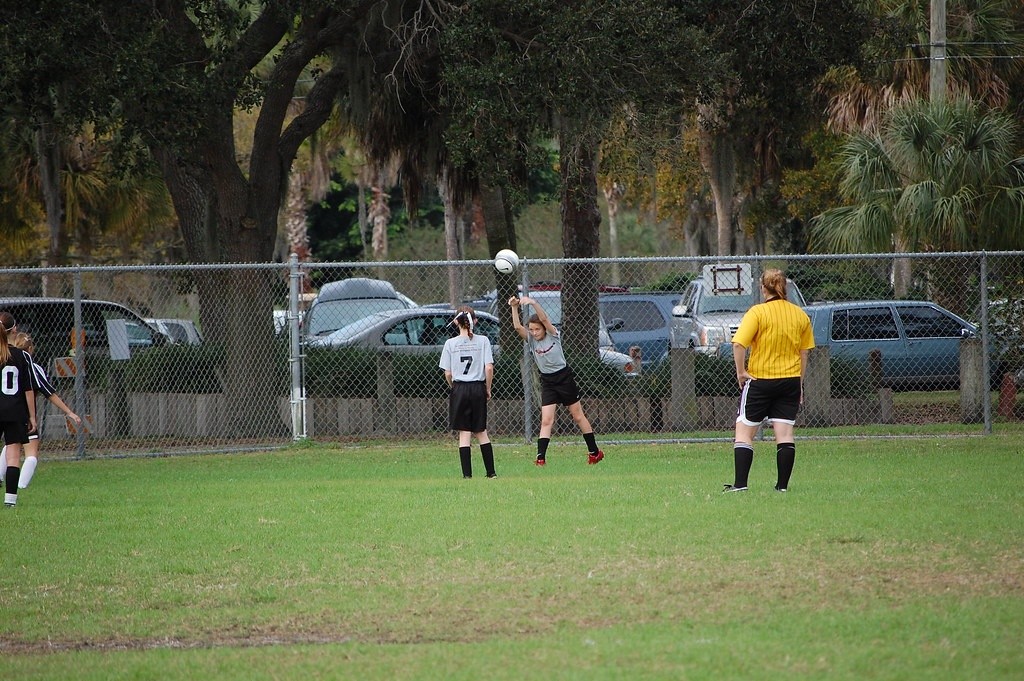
[
  {"x1": 485, "y1": 474, "x2": 496, "y2": 479},
  {"x1": 721, "y1": 484, "x2": 749, "y2": 495},
  {"x1": 773, "y1": 486, "x2": 786, "y2": 493},
  {"x1": 5, "y1": 503, "x2": 14, "y2": 510}
]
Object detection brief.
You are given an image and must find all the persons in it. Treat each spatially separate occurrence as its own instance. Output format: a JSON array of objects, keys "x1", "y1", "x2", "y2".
[
  {"x1": 444, "y1": 307, "x2": 499, "y2": 480},
  {"x1": 721, "y1": 269, "x2": 811, "y2": 494},
  {"x1": 509, "y1": 295, "x2": 605, "y2": 465},
  {"x1": 0, "y1": 311, "x2": 82, "y2": 508}
]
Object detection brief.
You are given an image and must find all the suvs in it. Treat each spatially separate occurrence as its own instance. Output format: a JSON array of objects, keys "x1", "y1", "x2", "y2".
[
  {"x1": 680, "y1": 276, "x2": 810, "y2": 359},
  {"x1": 799, "y1": 300, "x2": 1024, "y2": 391},
  {"x1": 0, "y1": 295, "x2": 190, "y2": 392}
]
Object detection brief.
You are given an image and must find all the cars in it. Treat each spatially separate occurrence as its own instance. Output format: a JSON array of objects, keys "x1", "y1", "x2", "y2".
[
  {"x1": 118, "y1": 315, "x2": 204, "y2": 347},
  {"x1": 269, "y1": 277, "x2": 683, "y2": 367},
  {"x1": 301, "y1": 309, "x2": 506, "y2": 356}
]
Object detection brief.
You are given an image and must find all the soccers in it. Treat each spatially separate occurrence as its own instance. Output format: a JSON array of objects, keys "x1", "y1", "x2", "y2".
[{"x1": 493, "y1": 248, "x2": 519, "y2": 275}]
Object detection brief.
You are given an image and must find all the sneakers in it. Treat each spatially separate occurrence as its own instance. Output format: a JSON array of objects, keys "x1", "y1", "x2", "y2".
[
  {"x1": 536, "y1": 460, "x2": 545, "y2": 466},
  {"x1": 588, "y1": 450, "x2": 603, "y2": 465}
]
